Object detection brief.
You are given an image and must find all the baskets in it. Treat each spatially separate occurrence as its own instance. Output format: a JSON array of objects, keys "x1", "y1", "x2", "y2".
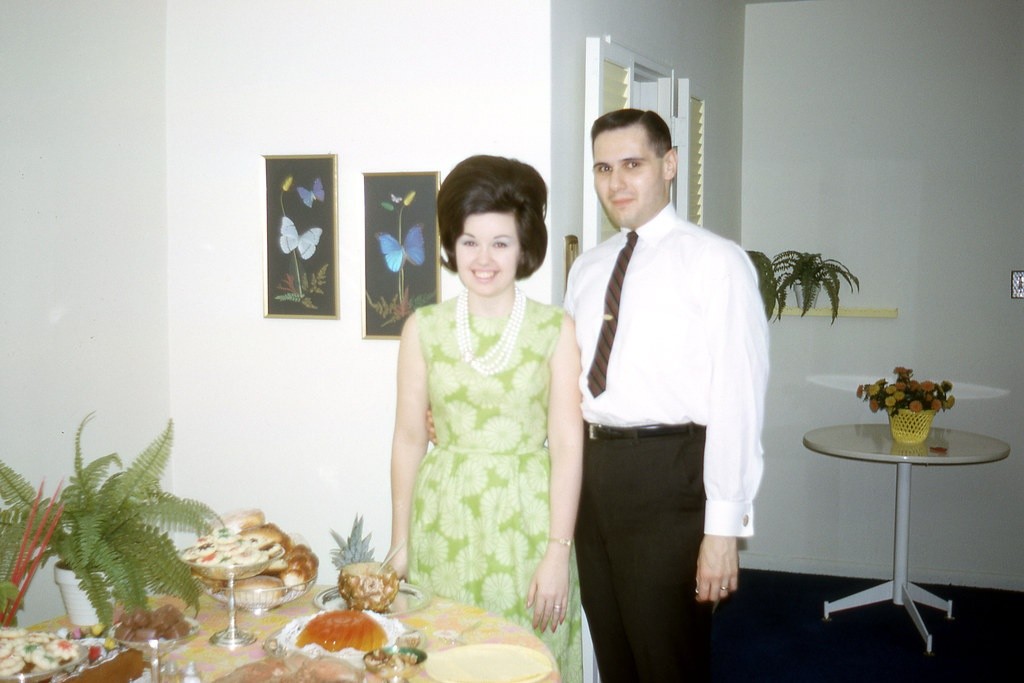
[{"x1": 886, "y1": 407, "x2": 936, "y2": 444}]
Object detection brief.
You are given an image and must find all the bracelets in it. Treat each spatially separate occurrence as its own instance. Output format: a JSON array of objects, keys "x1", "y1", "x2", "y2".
[{"x1": 548, "y1": 537, "x2": 573, "y2": 546}]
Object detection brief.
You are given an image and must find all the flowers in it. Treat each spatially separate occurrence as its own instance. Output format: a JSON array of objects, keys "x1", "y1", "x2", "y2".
[{"x1": 857, "y1": 367, "x2": 957, "y2": 416}]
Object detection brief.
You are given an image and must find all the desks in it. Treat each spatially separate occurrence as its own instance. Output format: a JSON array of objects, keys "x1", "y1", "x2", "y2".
[{"x1": 803, "y1": 423, "x2": 1011, "y2": 656}]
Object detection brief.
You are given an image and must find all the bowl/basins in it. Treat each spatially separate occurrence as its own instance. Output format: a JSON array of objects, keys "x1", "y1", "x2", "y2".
[{"x1": 198, "y1": 575, "x2": 318, "y2": 615}]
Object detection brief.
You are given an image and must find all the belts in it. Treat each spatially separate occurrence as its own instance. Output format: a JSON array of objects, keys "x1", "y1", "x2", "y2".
[{"x1": 587, "y1": 423, "x2": 689, "y2": 440}]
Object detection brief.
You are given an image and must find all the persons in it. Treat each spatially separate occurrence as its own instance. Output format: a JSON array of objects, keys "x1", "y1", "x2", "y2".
[
  {"x1": 561, "y1": 109, "x2": 770, "y2": 683},
  {"x1": 378, "y1": 154, "x2": 584, "y2": 683}
]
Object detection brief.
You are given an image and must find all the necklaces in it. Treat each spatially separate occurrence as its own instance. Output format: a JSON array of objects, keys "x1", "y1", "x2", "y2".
[{"x1": 456, "y1": 285, "x2": 527, "y2": 375}]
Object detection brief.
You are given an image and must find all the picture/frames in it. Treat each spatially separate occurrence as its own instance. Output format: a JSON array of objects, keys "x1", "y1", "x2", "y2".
[
  {"x1": 262, "y1": 155, "x2": 339, "y2": 321},
  {"x1": 362, "y1": 171, "x2": 439, "y2": 339}
]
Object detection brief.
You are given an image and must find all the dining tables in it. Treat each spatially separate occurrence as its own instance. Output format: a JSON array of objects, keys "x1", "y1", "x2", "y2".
[{"x1": 1, "y1": 575, "x2": 557, "y2": 682}]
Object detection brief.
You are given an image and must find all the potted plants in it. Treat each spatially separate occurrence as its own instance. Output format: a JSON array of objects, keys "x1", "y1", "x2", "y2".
[{"x1": 1, "y1": 408, "x2": 223, "y2": 625}]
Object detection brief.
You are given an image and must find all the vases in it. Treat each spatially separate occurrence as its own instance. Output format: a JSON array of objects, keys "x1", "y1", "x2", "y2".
[{"x1": 887, "y1": 409, "x2": 938, "y2": 445}]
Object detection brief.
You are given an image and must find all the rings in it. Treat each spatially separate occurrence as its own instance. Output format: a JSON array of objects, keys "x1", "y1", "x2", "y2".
[
  {"x1": 552, "y1": 604, "x2": 561, "y2": 612},
  {"x1": 720, "y1": 587, "x2": 729, "y2": 591}
]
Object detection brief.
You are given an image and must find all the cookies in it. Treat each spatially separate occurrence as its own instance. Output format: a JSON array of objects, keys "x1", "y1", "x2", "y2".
[
  {"x1": 179, "y1": 527, "x2": 269, "y2": 567},
  {"x1": 0, "y1": 626, "x2": 78, "y2": 679}
]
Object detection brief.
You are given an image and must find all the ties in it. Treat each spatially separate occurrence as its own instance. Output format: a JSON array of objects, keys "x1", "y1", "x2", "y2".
[{"x1": 588, "y1": 232, "x2": 638, "y2": 398}]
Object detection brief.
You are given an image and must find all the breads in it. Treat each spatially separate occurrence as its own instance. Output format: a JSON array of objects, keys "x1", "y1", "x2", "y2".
[
  {"x1": 212, "y1": 654, "x2": 364, "y2": 683},
  {"x1": 193, "y1": 509, "x2": 319, "y2": 605},
  {"x1": 112, "y1": 603, "x2": 189, "y2": 641}
]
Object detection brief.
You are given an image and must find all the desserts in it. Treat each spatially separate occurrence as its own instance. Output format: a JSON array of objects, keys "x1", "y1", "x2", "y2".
[{"x1": 275, "y1": 608, "x2": 406, "y2": 664}]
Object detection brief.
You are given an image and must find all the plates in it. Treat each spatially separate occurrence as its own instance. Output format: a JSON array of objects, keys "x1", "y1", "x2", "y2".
[
  {"x1": 314, "y1": 585, "x2": 426, "y2": 617},
  {"x1": 425, "y1": 643, "x2": 553, "y2": 683},
  {"x1": 262, "y1": 622, "x2": 426, "y2": 663},
  {"x1": 0, "y1": 643, "x2": 88, "y2": 683}
]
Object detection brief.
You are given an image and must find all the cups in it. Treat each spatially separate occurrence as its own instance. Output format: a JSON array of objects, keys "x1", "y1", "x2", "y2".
[{"x1": 928, "y1": 427, "x2": 951, "y2": 454}]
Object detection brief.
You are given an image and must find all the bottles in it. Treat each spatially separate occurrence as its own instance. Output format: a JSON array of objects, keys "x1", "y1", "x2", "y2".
[{"x1": 163, "y1": 661, "x2": 201, "y2": 683}]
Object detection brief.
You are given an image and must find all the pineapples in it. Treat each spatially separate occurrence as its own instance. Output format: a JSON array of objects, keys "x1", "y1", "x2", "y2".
[{"x1": 337, "y1": 562, "x2": 399, "y2": 611}]
